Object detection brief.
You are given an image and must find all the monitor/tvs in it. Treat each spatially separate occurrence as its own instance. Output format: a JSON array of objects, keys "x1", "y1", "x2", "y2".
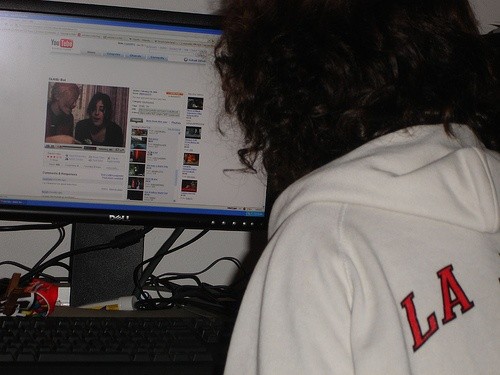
[{"x1": 1, "y1": 0, "x2": 278, "y2": 232}]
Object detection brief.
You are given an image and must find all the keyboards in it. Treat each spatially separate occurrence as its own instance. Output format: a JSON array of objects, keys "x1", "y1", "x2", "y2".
[{"x1": 0, "y1": 316, "x2": 238, "y2": 375}]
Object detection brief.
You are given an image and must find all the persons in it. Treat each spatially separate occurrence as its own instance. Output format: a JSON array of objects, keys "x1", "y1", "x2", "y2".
[
  {"x1": 208, "y1": 0, "x2": 500, "y2": 375},
  {"x1": 44, "y1": 82, "x2": 81, "y2": 145},
  {"x1": 75, "y1": 91, "x2": 123, "y2": 148}
]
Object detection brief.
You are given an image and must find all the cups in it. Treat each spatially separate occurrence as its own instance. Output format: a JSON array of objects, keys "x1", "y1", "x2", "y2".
[{"x1": 22, "y1": 279, "x2": 58, "y2": 317}]
